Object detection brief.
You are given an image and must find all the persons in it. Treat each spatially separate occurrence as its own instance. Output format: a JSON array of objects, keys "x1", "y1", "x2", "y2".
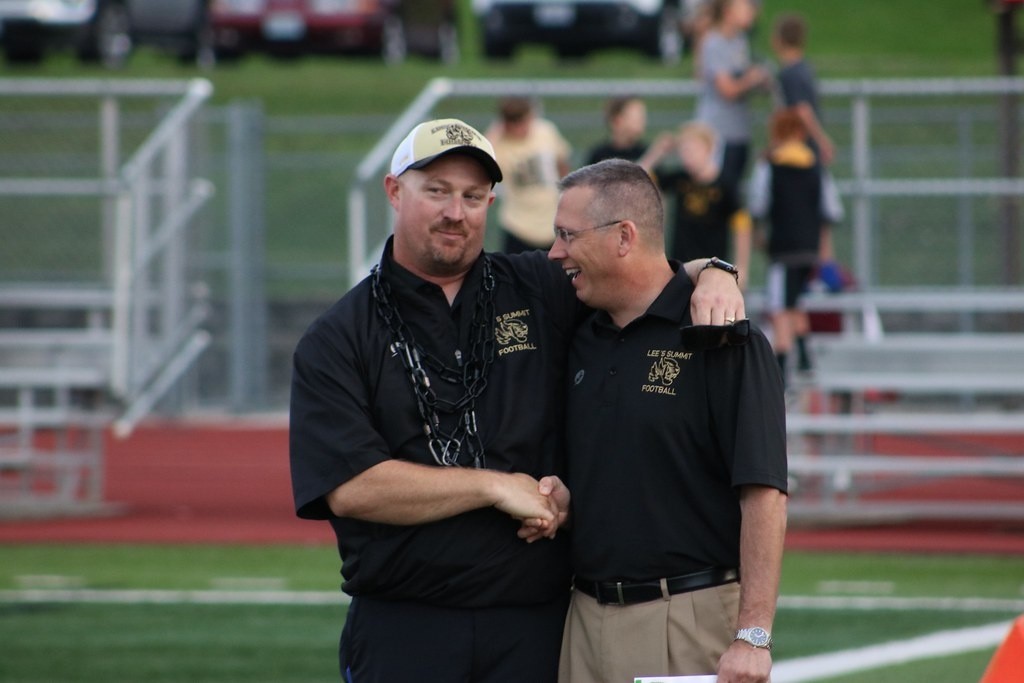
[
  {"x1": 289, "y1": 117, "x2": 745, "y2": 683},
  {"x1": 515, "y1": 159, "x2": 787, "y2": 683},
  {"x1": 473, "y1": 2, "x2": 840, "y2": 391}
]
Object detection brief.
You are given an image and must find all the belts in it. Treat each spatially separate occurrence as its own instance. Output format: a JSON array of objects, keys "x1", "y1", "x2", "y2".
[{"x1": 567, "y1": 570, "x2": 739, "y2": 606}]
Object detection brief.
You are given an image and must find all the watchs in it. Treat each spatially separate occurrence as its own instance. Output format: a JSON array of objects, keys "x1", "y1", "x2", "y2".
[
  {"x1": 696, "y1": 256, "x2": 739, "y2": 285},
  {"x1": 732, "y1": 626, "x2": 774, "y2": 651}
]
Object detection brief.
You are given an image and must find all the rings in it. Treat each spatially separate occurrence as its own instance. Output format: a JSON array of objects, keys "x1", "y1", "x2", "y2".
[{"x1": 724, "y1": 318, "x2": 734, "y2": 323}]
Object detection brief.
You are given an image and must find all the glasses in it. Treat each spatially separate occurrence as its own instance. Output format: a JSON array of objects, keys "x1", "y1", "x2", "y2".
[{"x1": 554, "y1": 217, "x2": 625, "y2": 242}]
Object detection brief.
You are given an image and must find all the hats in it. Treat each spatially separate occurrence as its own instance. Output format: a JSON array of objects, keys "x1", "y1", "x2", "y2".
[{"x1": 390, "y1": 118, "x2": 505, "y2": 182}]
